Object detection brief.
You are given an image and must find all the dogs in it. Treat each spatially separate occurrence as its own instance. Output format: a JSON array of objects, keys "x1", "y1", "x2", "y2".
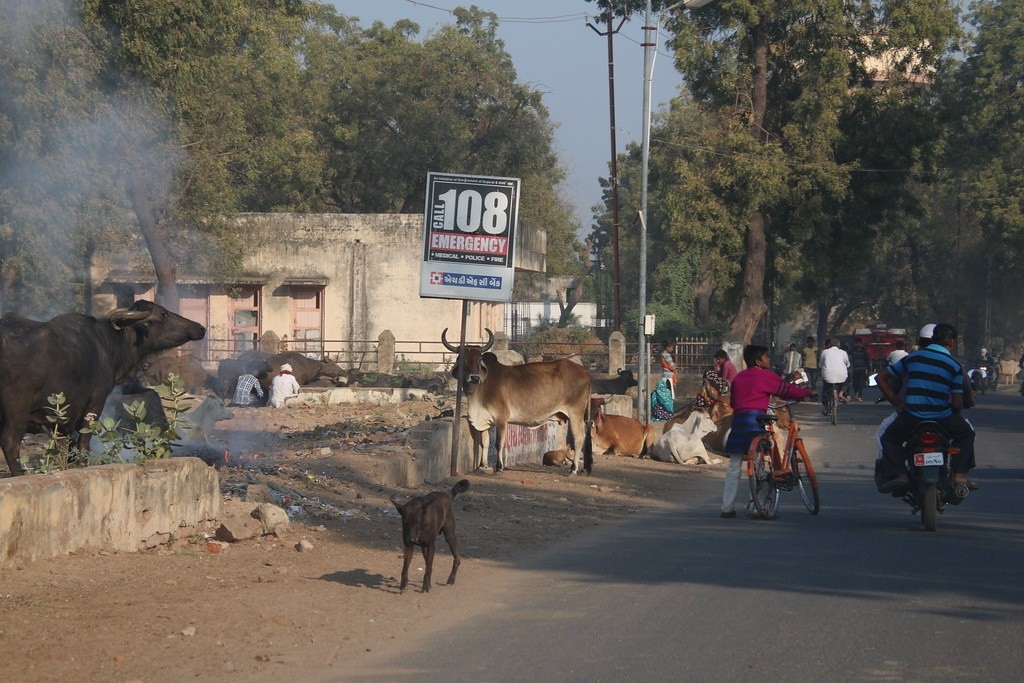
[{"x1": 390, "y1": 479, "x2": 471, "y2": 594}]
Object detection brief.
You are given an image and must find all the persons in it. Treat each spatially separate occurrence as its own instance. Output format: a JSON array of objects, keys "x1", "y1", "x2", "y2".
[
  {"x1": 852, "y1": 338, "x2": 873, "y2": 402},
  {"x1": 658, "y1": 342, "x2": 681, "y2": 402},
  {"x1": 819, "y1": 336, "x2": 851, "y2": 424},
  {"x1": 714, "y1": 348, "x2": 740, "y2": 383},
  {"x1": 1018, "y1": 348, "x2": 1024, "y2": 397},
  {"x1": 233, "y1": 366, "x2": 263, "y2": 406},
  {"x1": 782, "y1": 344, "x2": 802, "y2": 378},
  {"x1": 267, "y1": 364, "x2": 304, "y2": 408},
  {"x1": 720, "y1": 343, "x2": 816, "y2": 519},
  {"x1": 801, "y1": 336, "x2": 819, "y2": 402},
  {"x1": 978, "y1": 346, "x2": 994, "y2": 369},
  {"x1": 875, "y1": 324, "x2": 978, "y2": 495}
]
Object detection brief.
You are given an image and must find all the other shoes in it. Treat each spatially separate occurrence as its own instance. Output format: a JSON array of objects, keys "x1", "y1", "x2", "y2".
[
  {"x1": 847, "y1": 396, "x2": 851, "y2": 401},
  {"x1": 838, "y1": 395, "x2": 848, "y2": 403},
  {"x1": 719, "y1": 509, "x2": 738, "y2": 519},
  {"x1": 821, "y1": 408, "x2": 828, "y2": 416},
  {"x1": 856, "y1": 392, "x2": 858, "y2": 399},
  {"x1": 747, "y1": 500, "x2": 758, "y2": 509},
  {"x1": 858, "y1": 397, "x2": 863, "y2": 402}
]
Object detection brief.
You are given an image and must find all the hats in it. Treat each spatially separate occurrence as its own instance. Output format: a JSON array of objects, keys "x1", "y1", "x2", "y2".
[{"x1": 280, "y1": 363, "x2": 292, "y2": 372}]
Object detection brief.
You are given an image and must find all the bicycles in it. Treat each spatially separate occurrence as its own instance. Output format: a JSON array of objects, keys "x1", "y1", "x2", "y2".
[
  {"x1": 824, "y1": 390, "x2": 846, "y2": 426},
  {"x1": 747, "y1": 398, "x2": 820, "y2": 521}
]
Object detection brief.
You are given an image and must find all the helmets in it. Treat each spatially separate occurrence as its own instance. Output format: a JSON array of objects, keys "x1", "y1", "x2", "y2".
[
  {"x1": 919, "y1": 323, "x2": 936, "y2": 338},
  {"x1": 934, "y1": 323, "x2": 957, "y2": 340}
]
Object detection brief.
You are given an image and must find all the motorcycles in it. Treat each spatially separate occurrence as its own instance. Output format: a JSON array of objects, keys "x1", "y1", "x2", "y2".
[
  {"x1": 789, "y1": 370, "x2": 806, "y2": 388},
  {"x1": 866, "y1": 369, "x2": 988, "y2": 533}
]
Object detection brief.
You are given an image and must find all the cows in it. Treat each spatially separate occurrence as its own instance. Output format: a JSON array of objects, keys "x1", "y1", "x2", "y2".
[
  {"x1": 98, "y1": 375, "x2": 237, "y2": 458},
  {"x1": 583, "y1": 393, "x2": 657, "y2": 460},
  {"x1": 666, "y1": 376, "x2": 801, "y2": 461},
  {"x1": 591, "y1": 367, "x2": 639, "y2": 394},
  {"x1": 543, "y1": 448, "x2": 583, "y2": 467},
  {"x1": 0, "y1": 299, "x2": 208, "y2": 480},
  {"x1": 442, "y1": 327, "x2": 593, "y2": 479},
  {"x1": 654, "y1": 409, "x2": 724, "y2": 465},
  {"x1": 143, "y1": 348, "x2": 447, "y2": 404}
]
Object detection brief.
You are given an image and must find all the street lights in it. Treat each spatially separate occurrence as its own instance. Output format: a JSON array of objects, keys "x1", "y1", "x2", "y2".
[{"x1": 639, "y1": 0, "x2": 710, "y2": 426}]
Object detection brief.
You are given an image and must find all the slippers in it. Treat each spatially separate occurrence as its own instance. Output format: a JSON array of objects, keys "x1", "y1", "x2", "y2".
[
  {"x1": 878, "y1": 476, "x2": 908, "y2": 493},
  {"x1": 953, "y1": 480, "x2": 979, "y2": 490}
]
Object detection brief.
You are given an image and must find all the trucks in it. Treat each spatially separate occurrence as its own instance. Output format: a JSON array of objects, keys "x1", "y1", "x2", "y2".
[{"x1": 815, "y1": 327, "x2": 908, "y2": 369}]
